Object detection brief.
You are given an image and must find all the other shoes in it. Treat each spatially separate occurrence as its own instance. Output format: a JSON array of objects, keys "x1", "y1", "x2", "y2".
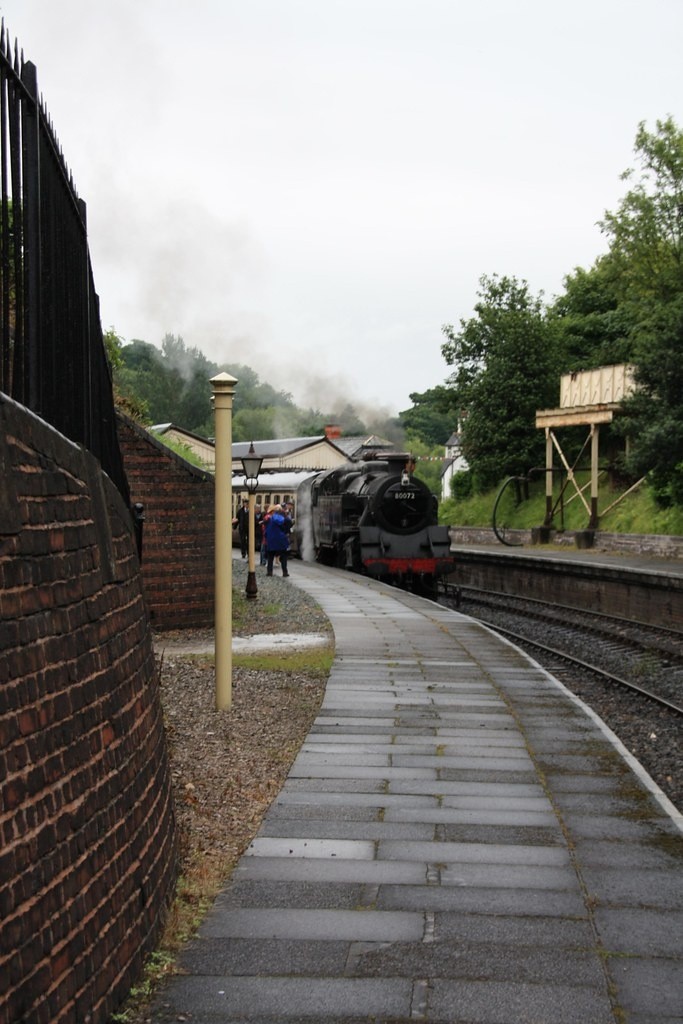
[
  {"x1": 283, "y1": 575, "x2": 289, "y2": 576},
  {"x1": 266, "y1": 574, "x2": 272, "y2": 576},
  {"x1": 242, "y1": 555, "x2": 245, "y2": 558},
  {"x1": 260, "y1": 563, "x2": 266, "y2": 565}
]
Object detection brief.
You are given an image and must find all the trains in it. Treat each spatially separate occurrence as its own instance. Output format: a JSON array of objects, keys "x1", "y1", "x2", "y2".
[{"x1": 233, "y1": 452, "x2": 455, "y2": 601}]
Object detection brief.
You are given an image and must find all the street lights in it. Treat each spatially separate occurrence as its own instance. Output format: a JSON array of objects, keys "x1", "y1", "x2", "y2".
[{"x1": 241, "y1": 441, "x2": 265, "y2": 603}]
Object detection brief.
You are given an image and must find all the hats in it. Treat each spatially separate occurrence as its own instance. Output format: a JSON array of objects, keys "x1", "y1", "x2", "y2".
[
  {"x1": 267, "y1": 504, "x2": 276, "y2": 513},
  {"x1": 244, "y1": 499, "x2": 249, "y2": 502},
  {"x1": 287, "y1": 499, "x2": 294, "y2": 505}
]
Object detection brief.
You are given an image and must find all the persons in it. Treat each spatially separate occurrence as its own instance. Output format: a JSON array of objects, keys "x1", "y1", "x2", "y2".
[
  {"x1": 260, "y1": 500, "x2": 296, "y2": 576},
  {"x1": 232, "y1": 499, "x2": 261, "y2": 558}
]
